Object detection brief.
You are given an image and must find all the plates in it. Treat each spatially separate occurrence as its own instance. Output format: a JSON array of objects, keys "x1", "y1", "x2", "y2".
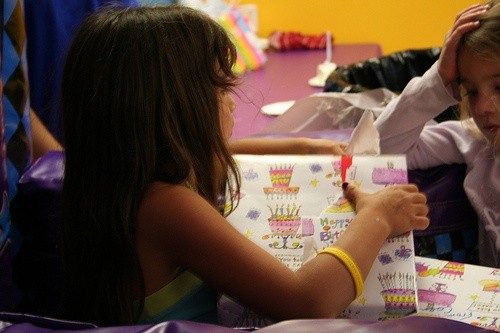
[
  {"x1": 261, "y1": 100, "x2": 297, "y2": 116},
  {"x1": 308, "y1": 76, "x2": 325, "y2": 87}
]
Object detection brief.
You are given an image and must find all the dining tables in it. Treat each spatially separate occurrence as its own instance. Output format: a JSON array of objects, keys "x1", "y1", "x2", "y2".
[{"x1": 229, "y1": 41, "x2": 383, "y2": 154}]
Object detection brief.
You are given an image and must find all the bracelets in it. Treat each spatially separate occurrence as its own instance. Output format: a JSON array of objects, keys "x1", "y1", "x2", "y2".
[{"x1": 317, "y1": 244, "x2": 366, "y2": 302}]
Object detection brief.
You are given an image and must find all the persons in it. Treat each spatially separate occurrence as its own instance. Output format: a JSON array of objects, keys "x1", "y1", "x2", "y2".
[
  {"x1": 325, "y1": 46, "x2": 477, "y2": 263},
  {"x1": 371, "y1": 0, "x2": 499, "y2": 270},
  {"x1": 20, "y1": 0, "x2": 148, "y2": 166},
  {"x1": 51, "y1": 3, "x2": 432, "y2": 325}
]
